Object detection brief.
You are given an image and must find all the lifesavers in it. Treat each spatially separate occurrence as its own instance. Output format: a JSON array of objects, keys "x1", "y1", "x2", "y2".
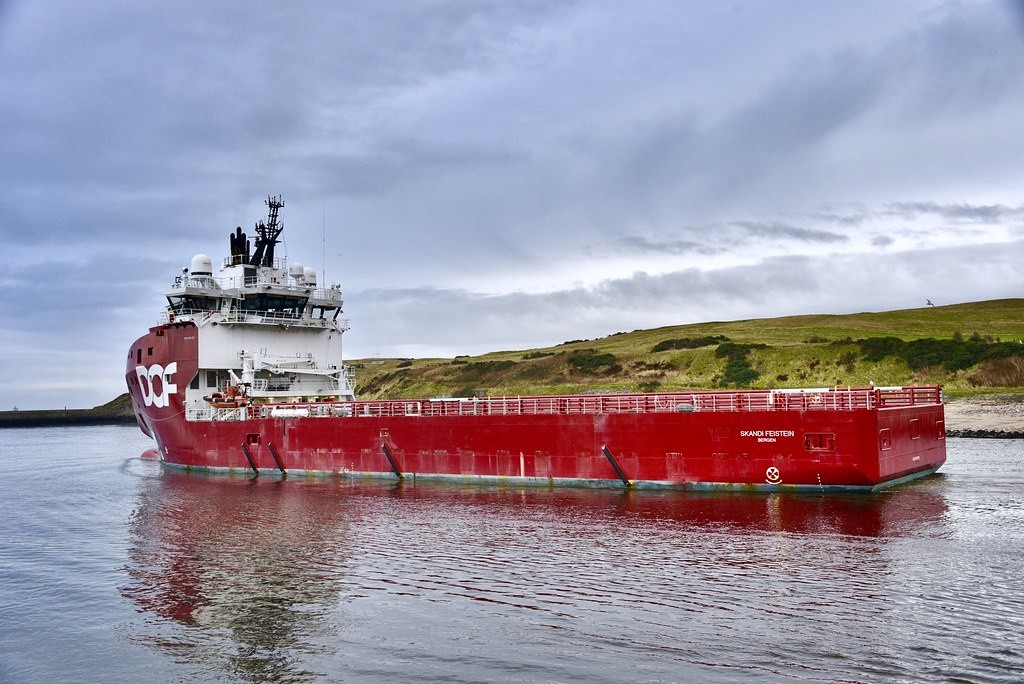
[
  {"x1": 208, "y1": 311, "x2": 214, "y2": 318},
  {"x1": 209, "y1": 393, "x2": 222, "y2": 407}
]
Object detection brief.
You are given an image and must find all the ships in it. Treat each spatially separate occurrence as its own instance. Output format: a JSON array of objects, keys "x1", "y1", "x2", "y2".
[{"x1": 124, "y1": 194, "x2": 948, "y2": 494}]
